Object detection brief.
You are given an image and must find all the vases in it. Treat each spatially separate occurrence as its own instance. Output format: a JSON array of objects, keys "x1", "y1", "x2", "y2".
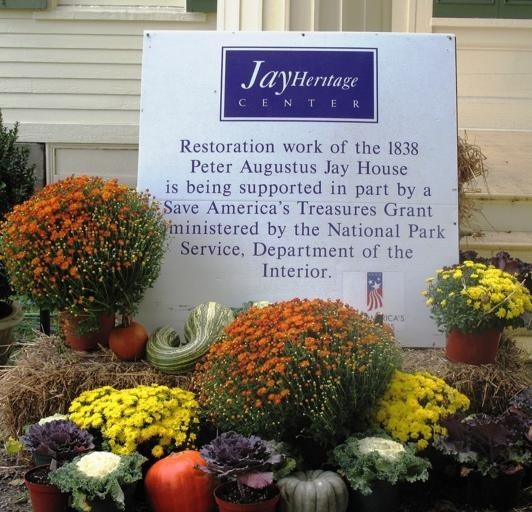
[
  {"x1": 63, "y1": 307, "x2": 116, "y2": 354},
  {"x1": 446, "y1": 322, "x2": 501, "y2": 363}
]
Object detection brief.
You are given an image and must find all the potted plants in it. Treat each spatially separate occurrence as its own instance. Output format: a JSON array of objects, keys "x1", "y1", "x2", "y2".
[
  {"x1": 319, "y1": 390, "x2": 532, "y2": 511},
  {"x1": 194, "y1": 429, "x2": 296, "y2": 511},
  {"x1": 0, "y1": 108, "x2": 37, "y2": 365},
  {"x1": 24, "y1": 420, "x2": 149, "y2": 512}
]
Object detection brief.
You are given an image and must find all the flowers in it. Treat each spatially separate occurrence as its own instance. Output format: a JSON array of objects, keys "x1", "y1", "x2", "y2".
[
  {"x1": 0, "y1": 173, "x2": 171, "y2": 337},
  {"x1": 420, "y1": 258, "x2": 532, "y2": 336},
  {"x1": 372, "y1": 362, "x2": 469, "y2": 450},
  {"x1": 70, "y1": 382, "x2": 202, "y2": 456},
  {"x1": 193, "y1": 299, "x2": 402, "y2": 443}
]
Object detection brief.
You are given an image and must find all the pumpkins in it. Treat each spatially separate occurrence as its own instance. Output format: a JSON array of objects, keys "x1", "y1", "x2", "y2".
[
  {"x1": 108, "y1": 314, "x2": 148, "y2": 361},
  {"x1": 145, "y1": 300, "x2": 235, "y2": 374},
  {"x1": 276, "y1": 469, "x2": 350, "y2": 512},
  {"x1": 143, "y1": 449, "x2": 215, "y2": 512}
]
